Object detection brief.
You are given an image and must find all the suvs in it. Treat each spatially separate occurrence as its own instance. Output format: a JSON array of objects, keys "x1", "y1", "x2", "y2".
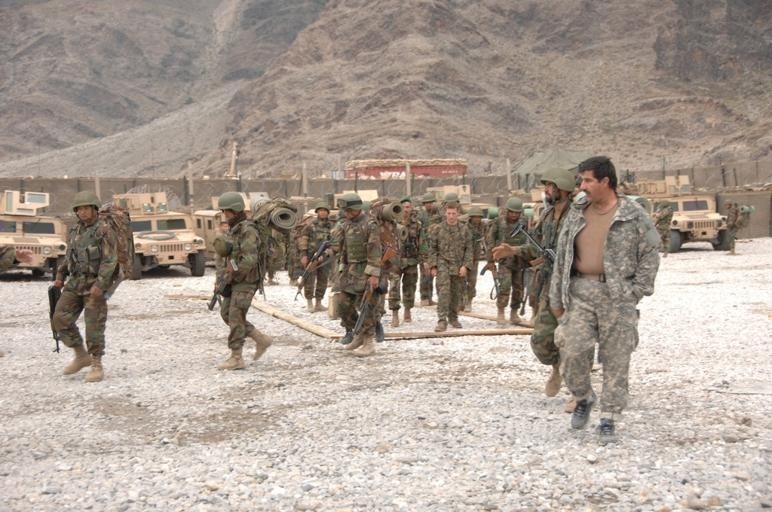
[
  {"x1": 113, "y1": 191, "x2": 207, "y2": 281},
  {"x1": 0, "y1": 190, "x2": 69, "y2": 285}
]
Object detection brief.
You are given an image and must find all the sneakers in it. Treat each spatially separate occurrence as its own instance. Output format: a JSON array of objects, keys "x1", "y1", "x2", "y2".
[
  {"x1": 570, "y1": 391, "x2": 598, "y2": 430},
  {"x1": 598, "y1": 418, "x2": 618, "y2": 446},
  {"x1": 544, "y1": 364, "x2": 563, "y2": 398},
  {"x1": 564, "y1": 394, "x2": 579, "y2": 414}
]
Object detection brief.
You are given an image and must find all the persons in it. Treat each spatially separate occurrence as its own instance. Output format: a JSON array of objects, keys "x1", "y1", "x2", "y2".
[
  {"x1": 723, "y1": 199, "x2": 738, "y2": 253},
  {"x1": 52, "y1": 191, "x2": 117, "y2": 381}
]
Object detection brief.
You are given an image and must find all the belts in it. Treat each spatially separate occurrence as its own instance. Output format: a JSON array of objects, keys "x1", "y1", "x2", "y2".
[{"x1": 574, "y1": 271, "x2": 607, "y2": 284}]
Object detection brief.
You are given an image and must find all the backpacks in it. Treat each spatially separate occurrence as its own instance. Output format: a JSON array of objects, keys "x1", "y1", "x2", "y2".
[
  {"x1": 733, "y1": 204, "x2": 756, "y2": 229},
  {"x1": 231, "y1": 195, "x2": 299, "y2": 267},
  {"x1": 364, "y1": 198, "x2": 403, "y2": 261}
]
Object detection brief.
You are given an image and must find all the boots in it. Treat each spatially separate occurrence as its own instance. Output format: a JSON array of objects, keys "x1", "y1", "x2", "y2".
[
  {"x1": 450, "y1": 318, "x2": 464, "y2": 329},
  {"x1": 419, "y1": 297, "x2": 438, "y2": 308},
  {"x1": 288, "y1": 278, "x2": 300, "y2": 287},
  {"x1": 403, "y1": 306, "x2": 412, "y2": 323},
  {"x1": 86, "y1": 354, "x2": 106, "y2": 383},
  {"x1": 62, "y1": 343, "x2": 93, "y2": 377},
  {"x1": 215, "y1": 346, "x2": 248, "y2": 371},
  {"x1": 391, "y1": 308, "x2": 400, "y2": 328},
  {"x1": 339, "y1": 322, "x2": 384, "y2": 358},
  {"x1": 434, "y1": 319, "x2": 449, "y2": 333},
  {"x1": 246, "y1": 327, "x2": 274, "y2": 362},
  {"x1": 267, "y1": 278, "x2": 280, "y2": 286},
  {"x1": 509, "y1": 308, "x2": 523, "y2": 324},
  {"x1": 497, "y1": 306, "x2": 507, "y2": 326},
  {"x1": 305, "y1": 297, "x2": 329, "y2": 315},
  {"x1": 726, "y1": 247, "x2": 736, "y2": 257},
  {"x1": 463, "y1": 298, "x2": 473, "y2": 313}
]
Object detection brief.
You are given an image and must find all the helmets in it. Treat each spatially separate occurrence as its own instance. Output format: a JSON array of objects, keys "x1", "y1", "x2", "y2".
[
  {"x1": 443, "y1": 192, "x2": 460, "y2": 203},
  {"x1": 723, "y1": 200, "x2": 733, "y2": 206},
  {"x1": 506, "y1": 197, "x2": 523, "y2": 213},
  {"x1": 217, "y1": 191, "x2": 246, "y2": 214},
  {"x1": 540, "y1": 166, "x2": 575, "y2": 193},
  {"x1": 467, "y1": 207, "x2": 484, "y2": 218},
  {"x1": 420, "y1": 192, "x2": 437, "y2": 203},
  {"x1": 70, "y1": 190, "x2": 103, "y2": 213},
  {"x1": 335, "y1": 192, "x2": 363, "y2": 211},
  {"x1": 314, "y1": 201, "x2": 331, "y2": 214}
]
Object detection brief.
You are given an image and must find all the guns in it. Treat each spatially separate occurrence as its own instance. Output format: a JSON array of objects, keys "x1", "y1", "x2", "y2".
[
  {"x1": 294, "y1": 241, "x2": 331, "y2": 299},
  {"x1": 48, "y1": 285, "x2": 62, "y2": 353},
  {"x1": 354, "y1": 248, "x2": 399, "y2": 335},
  {"x1": 206, "y1": 259, "x2": 239, "y2": 311},
  {"x1": 476, "y1": 237, "x2": 501, "y2": 294}
]
